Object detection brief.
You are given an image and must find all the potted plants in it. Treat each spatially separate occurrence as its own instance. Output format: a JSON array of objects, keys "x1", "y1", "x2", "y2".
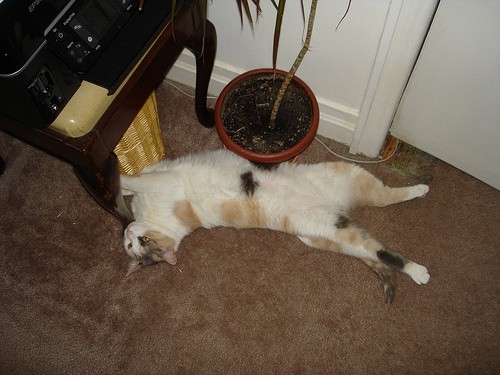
[{"x1": 214, "y1": 0, "x2": 351, "y2": 167}]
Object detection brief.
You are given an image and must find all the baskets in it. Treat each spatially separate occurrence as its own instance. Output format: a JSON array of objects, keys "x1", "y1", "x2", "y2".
[{"x1": 113, "y1": 90, "x2": 167, "y2": 177}]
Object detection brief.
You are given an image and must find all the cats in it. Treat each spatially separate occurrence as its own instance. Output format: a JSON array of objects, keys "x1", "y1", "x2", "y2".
[{"x1": 120, "y1": 147, "x2": 430, "y2": 304}]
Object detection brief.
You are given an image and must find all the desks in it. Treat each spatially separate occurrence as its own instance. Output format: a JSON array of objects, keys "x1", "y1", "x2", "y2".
[{"x1": 0, "y1": 0, "x2": 218, "y2": 232}]
